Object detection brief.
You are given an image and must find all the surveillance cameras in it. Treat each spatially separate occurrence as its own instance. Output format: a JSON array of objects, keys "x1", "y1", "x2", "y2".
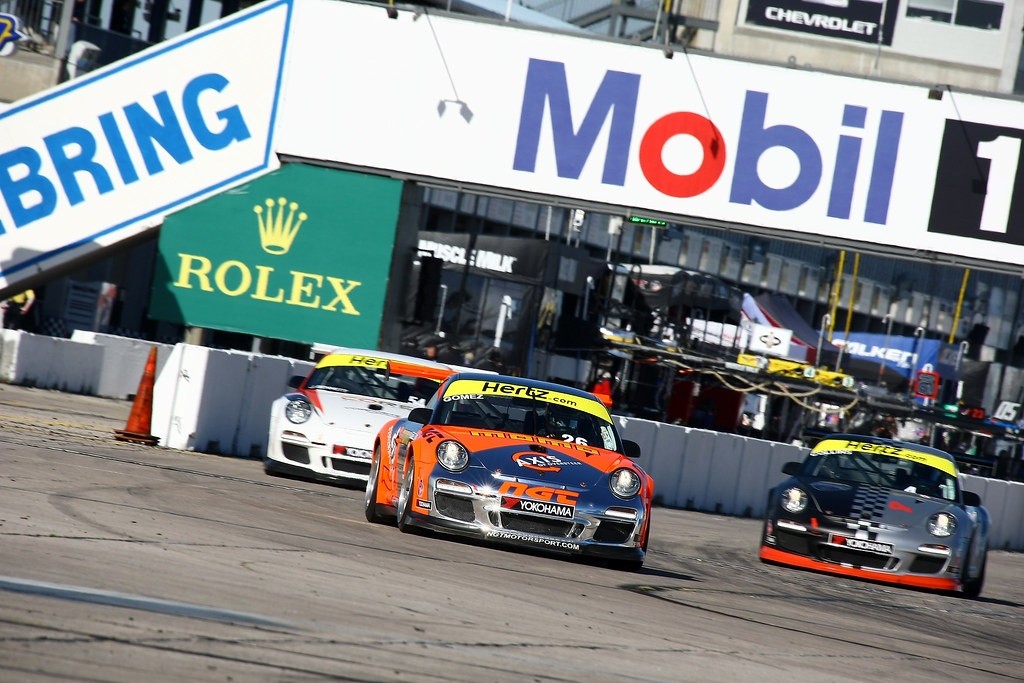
[{"x1": 572, "y1": 211, "x2": 585, "y2": 233}]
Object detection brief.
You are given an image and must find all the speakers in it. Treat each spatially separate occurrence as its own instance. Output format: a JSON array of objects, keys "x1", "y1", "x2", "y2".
[{"x1": 400, "y1": 256, "x2": 442, "y2": 322}]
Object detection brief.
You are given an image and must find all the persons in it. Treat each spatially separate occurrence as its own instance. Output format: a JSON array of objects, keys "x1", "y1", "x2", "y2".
[
  {"x1": 739, "y1": 409, "x2": 760, "y2": 439},
  {"x1": 537, "y1": 404, "x2": 575, "y2": 438},
  {"x1": 0, "y1": 288, "x2": 35, "y2": 331},
  {"x1": 868, "y1": 416, "x2": 897, "y2": 439}
]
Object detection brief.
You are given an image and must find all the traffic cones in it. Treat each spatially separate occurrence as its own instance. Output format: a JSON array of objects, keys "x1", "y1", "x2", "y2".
[{"x1": 113, "y1": 345, "x2": 162, "y2": 445}]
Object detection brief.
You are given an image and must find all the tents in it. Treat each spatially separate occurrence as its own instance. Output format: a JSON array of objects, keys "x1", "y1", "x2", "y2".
[{"x1": 735, "y1": 289, "x2": 853, "y2": 432}]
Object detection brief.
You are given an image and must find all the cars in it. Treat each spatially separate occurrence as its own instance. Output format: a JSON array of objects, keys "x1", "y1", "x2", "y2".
[
  {"x1": 757, "y1": 432, "x2": 992, "y2": 598},
  {"x1": 364, "y1": 370, "x2": 655, "y2": 571},
  {"x1": 261, "y1": 348, "x2": 458, "y2": 483}
]
